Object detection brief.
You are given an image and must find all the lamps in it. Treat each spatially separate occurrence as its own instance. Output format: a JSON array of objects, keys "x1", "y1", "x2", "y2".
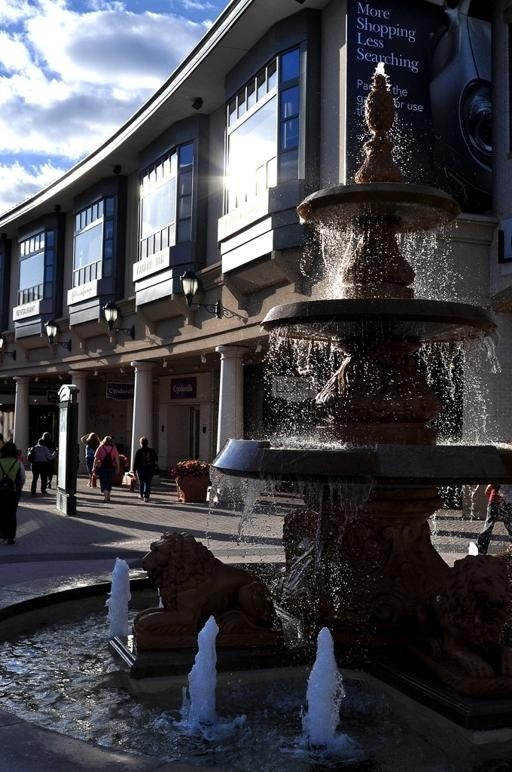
[
  {"x1": 0, "y1": 336, "x2": 16, "y2": 360},
  {"x1": 44, "y1": 318, "x2": 71, "y2": 351},
  {"x1": 179, "y1": 270, "x2": 221, "y2": 319},
  {"x1": 103, "y1": 300, "x2": 135, "y2": 340}
]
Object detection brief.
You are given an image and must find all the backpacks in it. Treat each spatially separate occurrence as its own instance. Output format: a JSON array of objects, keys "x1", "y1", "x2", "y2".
[
  {"x1": 98, "y1": 446, "x2": 116, "y2": 470},
  {"x1": 1, "y1": 460, "x2": 17, "y2": 516}
]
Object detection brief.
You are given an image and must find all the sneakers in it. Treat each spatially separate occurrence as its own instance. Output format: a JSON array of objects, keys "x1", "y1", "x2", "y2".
[
  {"x1": 138, "y1": 495, "x2": 149, "y2": 501},
  {"x1": 101, "y1": 500, "x2": 110, "y2": 503}
]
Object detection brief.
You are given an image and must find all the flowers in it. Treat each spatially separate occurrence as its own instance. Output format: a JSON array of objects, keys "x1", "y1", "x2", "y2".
[{"x1": 171, "y1": 460, "x2": 209, "y2": 477}]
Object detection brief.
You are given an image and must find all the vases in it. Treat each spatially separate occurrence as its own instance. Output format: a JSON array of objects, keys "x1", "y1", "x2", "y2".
[{"x1": 176, "y1": 475, "x2": 208, "y2": 503}]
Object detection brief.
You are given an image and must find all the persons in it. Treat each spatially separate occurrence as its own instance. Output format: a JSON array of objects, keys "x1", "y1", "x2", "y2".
[
  {"x1": 0, "y1": 430, "x2": 158, "y2": 546},
  {"x1": 475, "y1": 484, "x2": 512, "y2": 555}
]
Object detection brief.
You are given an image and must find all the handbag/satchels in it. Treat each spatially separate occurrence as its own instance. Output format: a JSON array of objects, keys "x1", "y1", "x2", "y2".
[
  {"x1": 90, "y1": 475, "x2": 96, "y2": 488},
  {"x1": 28, "y1": 449, "x2": 35, "y2": 463},
  {"x1": 152, "y1": 464, "x2": 162, "y2": 474}
]
